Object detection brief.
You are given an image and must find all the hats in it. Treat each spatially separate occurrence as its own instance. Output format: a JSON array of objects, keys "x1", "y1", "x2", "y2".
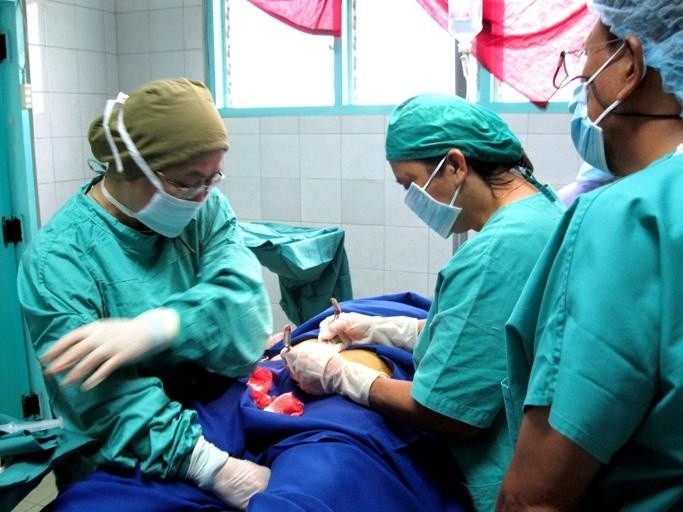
[
  {"x1": 88, "y1": 77, "x2": 228, "y2": 180},
  {"x1": 385, "y1": 92, "x2": 524, "y2": 162}
]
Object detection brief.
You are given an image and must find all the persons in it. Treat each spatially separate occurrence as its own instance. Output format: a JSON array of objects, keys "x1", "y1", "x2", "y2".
[
  {"x1": 12, "y1": 77, "x2": 277, "y2": 511},
  {"x1": 280, "y1": 91, "x2": 571, "y2": 511},
  {"x1": 497, "y1": 0, "x2": 683, "y2": 511}
]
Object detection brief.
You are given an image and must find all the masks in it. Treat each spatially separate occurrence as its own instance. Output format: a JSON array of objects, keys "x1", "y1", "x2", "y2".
[
  {"x1": 106, "y1": 151, "x2": 211, "y2": 239},
  {"x1": 404, "y1": 156, "x2": 463, "y2": 239},
  {"x1": 568, "y1": 39, "x2": 646, "y2": 176}
]
